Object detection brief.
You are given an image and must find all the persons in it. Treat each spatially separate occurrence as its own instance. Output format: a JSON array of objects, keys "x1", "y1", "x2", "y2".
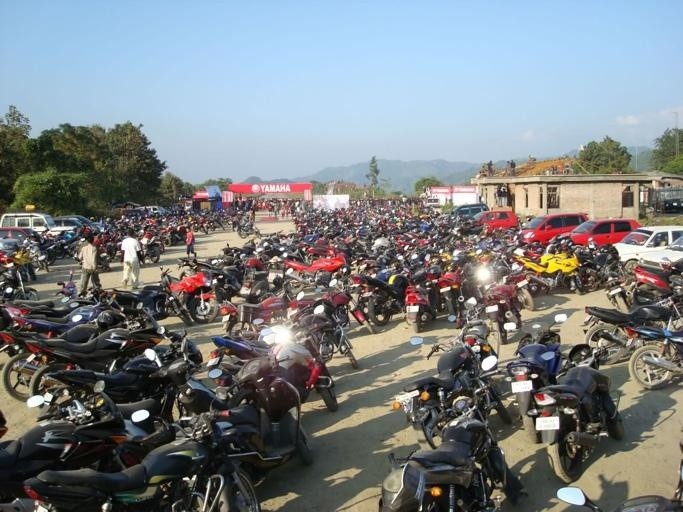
[
  {"x1": 77, "y1": 231, "x2": 102, "y2": 295},
  {"x1": 185, "y1": 227, "x2": 196, "y2": 257},
  {"x1": 120, "y1": 229, "x2": 144, "y2": 289}
]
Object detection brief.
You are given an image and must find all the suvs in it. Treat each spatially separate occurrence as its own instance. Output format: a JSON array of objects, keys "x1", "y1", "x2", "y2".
[{"x1": 652, "y1": 187, "x2": 683, "y2": 213}]
